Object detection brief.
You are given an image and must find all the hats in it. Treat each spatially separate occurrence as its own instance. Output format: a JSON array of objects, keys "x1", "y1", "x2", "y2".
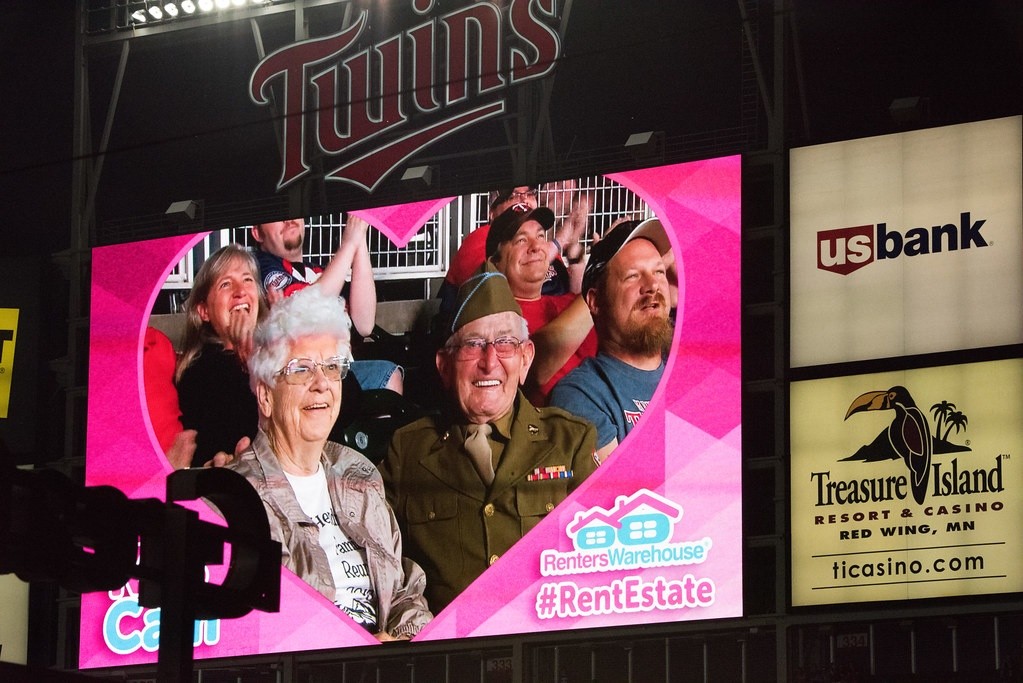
[
  {"x1": 489, "y1": 185, "x2": 535, "y2": 209},
  {"x1": 486, "y1": 203, "x2": 555, "y2": 260},
  {"x1": 582, "y1": 217, "x2": 672, "y2": 303},
  {"x1": 441, "y1": 271, "x2": 522, "y2": 349}
]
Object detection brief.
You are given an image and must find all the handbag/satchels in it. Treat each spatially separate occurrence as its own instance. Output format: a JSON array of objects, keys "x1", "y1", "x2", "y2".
[{"x1": 351, "y1": 323, "x2": 421, "y2": 382}]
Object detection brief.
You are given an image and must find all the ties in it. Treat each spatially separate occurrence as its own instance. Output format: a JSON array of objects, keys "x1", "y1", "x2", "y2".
[{"x1": 464, "y1": 424, "x2": 495, "y2": 491}]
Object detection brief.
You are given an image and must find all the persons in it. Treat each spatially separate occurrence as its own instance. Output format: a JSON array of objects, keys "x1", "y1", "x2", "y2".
[{"x1": 141, "y1": 182, "x2": 679, "y2": 641}]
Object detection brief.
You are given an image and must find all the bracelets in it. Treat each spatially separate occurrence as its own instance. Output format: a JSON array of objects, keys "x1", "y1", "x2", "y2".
[
  {"x1": 553, "y1": 238, "x2": 561, "y2": 250},
  {"x1": 568, "y1": 249, "x2": 585, "y2": 265}
]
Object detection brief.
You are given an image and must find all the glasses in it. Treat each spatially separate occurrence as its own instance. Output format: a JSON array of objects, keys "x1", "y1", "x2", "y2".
[
  {"x1": 272, "y1": 354, "x2": 350, "y2": 385},
  {"x1": 503, "y1": 188, "x2": 535, "y2": 203},
  {"x1": 444, "y1": 337, "x2": 524, "y2": 361}
]
